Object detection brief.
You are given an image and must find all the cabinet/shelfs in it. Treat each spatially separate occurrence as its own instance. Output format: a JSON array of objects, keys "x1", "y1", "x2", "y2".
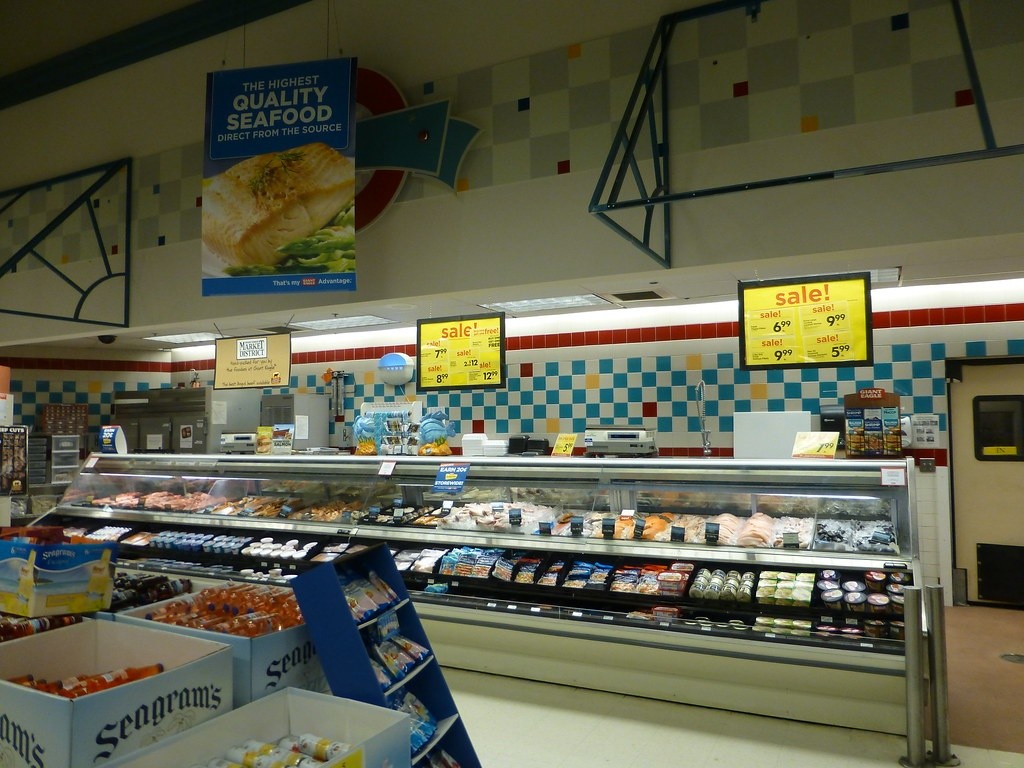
[
  {"x1": 290, "y1": 541, "x2": 483, "y2": 768},
  {"x1": 59, "y1": 451, "x2": 924, "y2": 671}
]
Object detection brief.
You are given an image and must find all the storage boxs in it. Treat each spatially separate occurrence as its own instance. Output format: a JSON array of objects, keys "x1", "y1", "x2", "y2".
[
  {"x1": 900, "y1": 413, "x2": 938, "y2": 450},
  {"x1": 9, "y1": 434, "x2": 80, "y2": 518},
  {"x1": 0, "y1": 539, "x2": 411, "y2": 768}
]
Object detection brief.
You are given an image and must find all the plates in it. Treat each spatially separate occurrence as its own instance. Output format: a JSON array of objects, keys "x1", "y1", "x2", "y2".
[{"x1": 201, "y1": 157, "x2": 354, "y2": 277}]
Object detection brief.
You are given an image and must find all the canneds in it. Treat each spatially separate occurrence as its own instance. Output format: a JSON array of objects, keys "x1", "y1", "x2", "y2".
[{"x1": 187, "y1": 732, "x2": 356, "y2": 768}]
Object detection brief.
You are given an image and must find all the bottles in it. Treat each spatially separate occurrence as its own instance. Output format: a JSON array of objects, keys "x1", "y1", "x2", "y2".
[
  {"x1": 0, "y1": 612, "x2": 82, "y2": 642},
  {"x1": 16, "y1": 550, "x2": 37, "y2": 610},
  {"x1": 9, "y1": 664, "x2": 164, "y2": 697},
  {"x1": 87, "y1": 550, "x2": 111, "y2": 610},
  {"x1": 108, "y1": 573, "x2": 305, "y2": 638}
]
